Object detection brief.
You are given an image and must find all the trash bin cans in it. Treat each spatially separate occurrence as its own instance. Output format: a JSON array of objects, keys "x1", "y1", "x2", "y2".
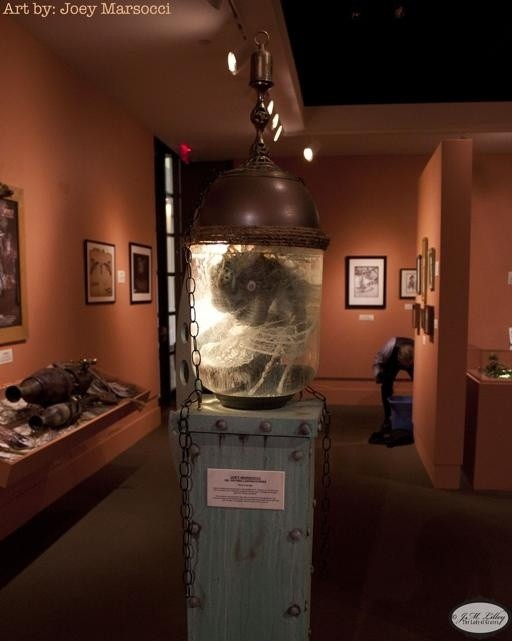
[{"x1": 387, "y1": 396, "x2": 411, "y2": 430}]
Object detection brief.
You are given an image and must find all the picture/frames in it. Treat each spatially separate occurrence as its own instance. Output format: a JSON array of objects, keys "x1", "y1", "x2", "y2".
[
  {"x1": 83, "y1": 234, "x2": 153, "y2": 305},
  {"x1": 343, "y1": 253, "x2": 418, "y2": 309},
  {"x1": 1, "y1": 183, "x2": 30, "y2": 345},
  {"x1": 412, "y1": 238, "x2": 436, "y2": 336}
]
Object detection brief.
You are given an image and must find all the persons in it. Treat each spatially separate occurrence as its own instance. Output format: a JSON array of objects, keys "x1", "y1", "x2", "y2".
[
  {"x1": 369, "y1": 334, "x2": 415, "y2": 444},
  {"x1": 360, "y1": 272, "x2": 367, "y2": 287},
  {"x1": 409, "y1": 276, "x2": 414, "y2": 289}
]
